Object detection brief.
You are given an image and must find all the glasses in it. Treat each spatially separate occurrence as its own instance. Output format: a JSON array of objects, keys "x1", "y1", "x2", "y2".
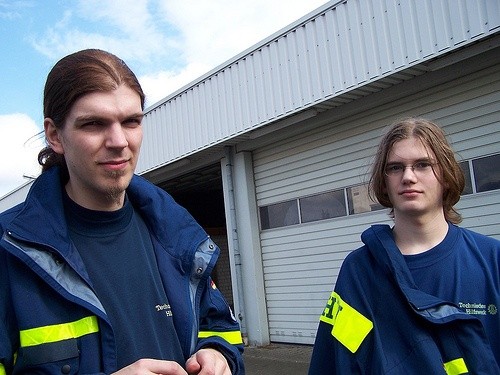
[{"x1": 384, "y1": 161, "x2": 439, "y2": 177}]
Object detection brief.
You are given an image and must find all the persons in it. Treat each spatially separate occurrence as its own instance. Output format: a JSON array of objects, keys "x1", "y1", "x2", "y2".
[
  {"x1": 307, "y1": 117, "x2": 500, "y2": 375},
  {"x1": 0, "y1": 47, "x2": 248, "y2": 375}
]
